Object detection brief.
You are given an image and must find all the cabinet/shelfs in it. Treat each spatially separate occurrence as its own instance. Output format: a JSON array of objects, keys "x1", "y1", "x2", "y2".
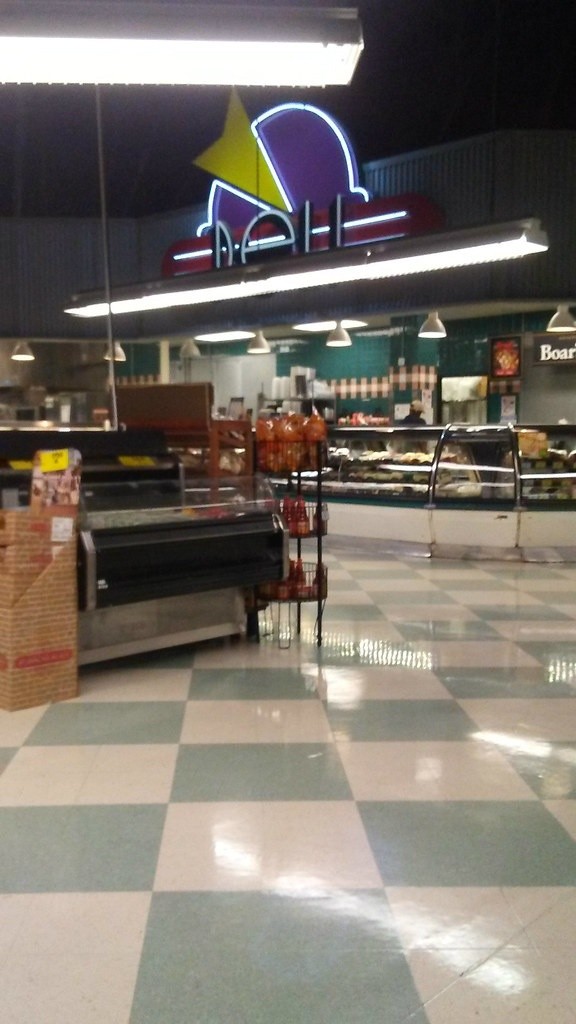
[{"x1": 247, "y1": 439, "x2": 331, "y2": 646}]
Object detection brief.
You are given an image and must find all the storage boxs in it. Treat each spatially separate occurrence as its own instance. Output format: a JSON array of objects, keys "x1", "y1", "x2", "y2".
[{"x1": 0, "y1": 505, "x2": 79, "y2": 711}]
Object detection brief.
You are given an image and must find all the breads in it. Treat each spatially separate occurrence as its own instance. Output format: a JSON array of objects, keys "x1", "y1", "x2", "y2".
[{"x1": 256, "y1": 415, "x2": 327, "y2": 471}]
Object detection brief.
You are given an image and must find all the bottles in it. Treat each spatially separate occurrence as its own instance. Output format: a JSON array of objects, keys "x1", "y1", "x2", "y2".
[
  {"x1": 283, "y1": 495, "x2": 310, "y2": 535},
  {"x1": 279, "y1": 559, "x2": 305, "y2": 598}
]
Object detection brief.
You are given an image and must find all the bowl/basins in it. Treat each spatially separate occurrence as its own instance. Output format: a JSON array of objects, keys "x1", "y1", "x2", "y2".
[
  {"x1": 281, "y1": 401, "x2": 301, "y2": 413},
  {"x1": 271, "y1": 367, "x2": 303, "y2": 397}
]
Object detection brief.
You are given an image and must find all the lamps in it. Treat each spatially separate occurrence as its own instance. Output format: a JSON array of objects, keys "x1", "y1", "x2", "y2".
[
  {"x1": 11, "y1": 340, "x2": 36, "y2": 361},
  {"x1": 247, "y1": 329, "x2": 271, "y2": 353},
  {"x1": 104, "y1": 342, "x2": 127, "y2": 361},
  {"x1": 326, "y1": 319, "x2": 352, "y2": 347},
  {"x1": 65, "y1": 217, "x2": 550, "y2": 319},
  {"x1": 546, "y1": 304, "x2": 576, "y2": 332},
  {"x1": 179, "y1": 336, "x2": 201, "y2": 359},
  {"x1": 418, "y1": 311, "x2": 447, "y2": 339}
]
